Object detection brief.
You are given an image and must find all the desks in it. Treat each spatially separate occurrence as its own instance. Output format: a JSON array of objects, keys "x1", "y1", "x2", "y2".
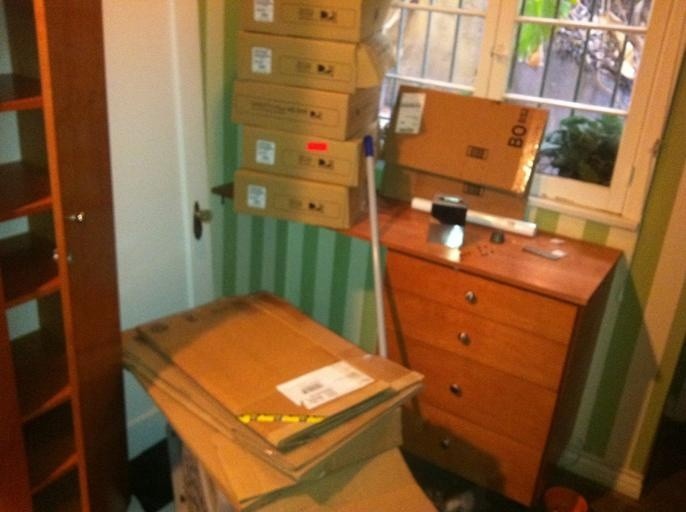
[{"x1": 208, "y1": 175, "x2": 624, "y2": 509}]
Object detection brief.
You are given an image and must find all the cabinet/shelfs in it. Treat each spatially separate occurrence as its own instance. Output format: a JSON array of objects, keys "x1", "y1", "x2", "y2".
[{"x1": 0, "y1": 2, "x2": 144, "y2": 512}]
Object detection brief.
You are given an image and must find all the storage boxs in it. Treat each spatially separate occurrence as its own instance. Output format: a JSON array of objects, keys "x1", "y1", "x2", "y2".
[{"x1": 226, "y1": 1, "x2": 398, "y2": 233}]
